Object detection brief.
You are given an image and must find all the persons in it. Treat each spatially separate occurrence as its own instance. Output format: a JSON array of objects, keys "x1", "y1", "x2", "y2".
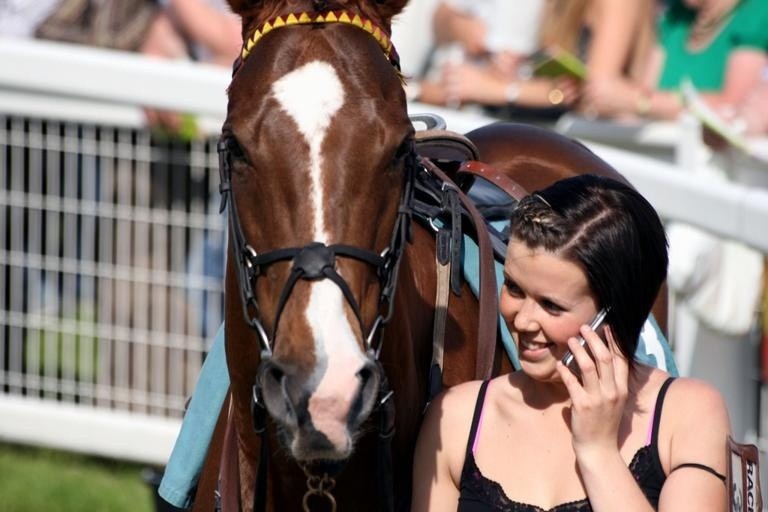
[{"x1": 0, "y1": 0, "x2": 767, "y2": 512}]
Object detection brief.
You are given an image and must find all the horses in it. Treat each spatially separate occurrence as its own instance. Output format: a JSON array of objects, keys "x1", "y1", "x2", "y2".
[{"x1": 190, "y1": 0, "x2": 668, "y2": 512}]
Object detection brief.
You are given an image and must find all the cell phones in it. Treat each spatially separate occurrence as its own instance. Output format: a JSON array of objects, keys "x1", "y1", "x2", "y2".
[{"x1": 561, "y1": 306, "x2": 614, "y2": 379}]
